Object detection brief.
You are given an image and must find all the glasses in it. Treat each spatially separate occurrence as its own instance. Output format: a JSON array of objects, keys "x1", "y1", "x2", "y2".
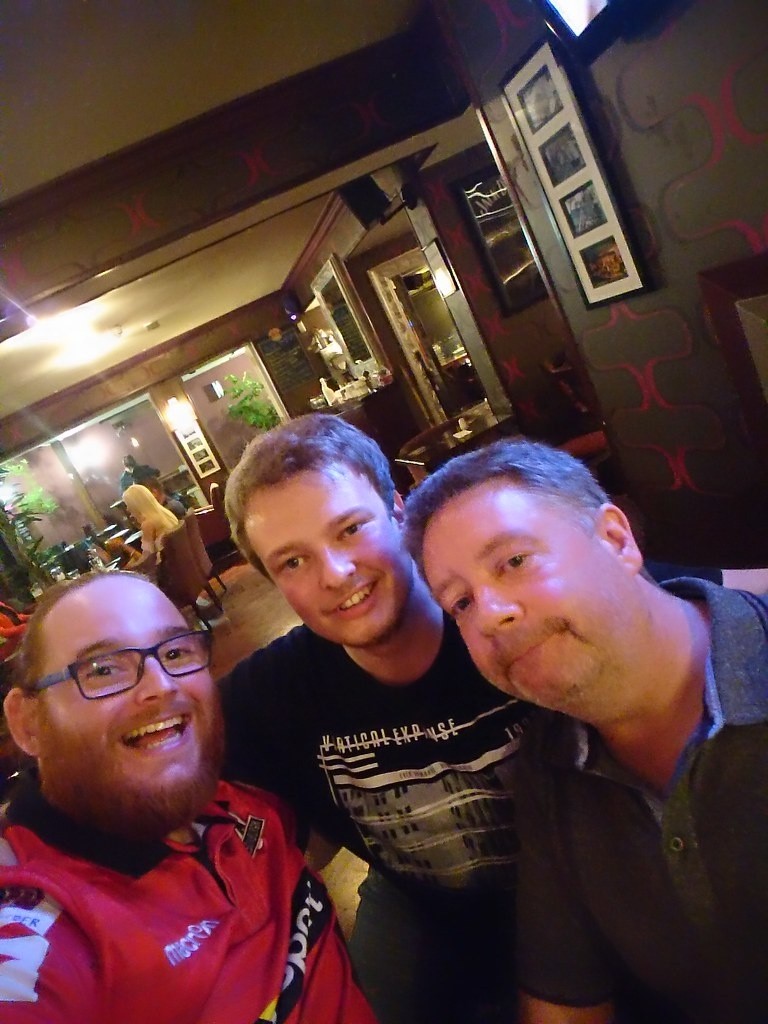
[{"x1": 27, "y1": 630, "x2": 212, "y2": 702}]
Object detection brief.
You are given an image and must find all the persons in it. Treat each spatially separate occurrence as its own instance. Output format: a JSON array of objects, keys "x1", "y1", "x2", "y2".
[
  {"x1": 399, "y1": 435, "x2": 768, "y2": 1024},
  {"x1": 118, "y1": 455, "x2": 160, "y2": 496},
  {"x1": 0, "y1": 601, "x2": 34, "y2": 642},
  {"x1": 139, "y1": 476, "x2": 186, "y2": 519},
  {"x1": 0, "y1": 569, "x2": 381, "y2": 1024},
  {"x1": 0, "y1": 412, "x2": 768, "y2": 1024},
  {"x1": 104, "y1": 485, "x2": 178, "y2": 565}
]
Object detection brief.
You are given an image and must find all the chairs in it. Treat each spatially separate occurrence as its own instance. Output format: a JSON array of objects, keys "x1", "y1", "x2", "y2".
[
  {"x1": 192, "y1": 479, "x2": 234, "y2": 550},
  {"x1": 150, "y1": 508, "x2": 230, "y2": 620}
]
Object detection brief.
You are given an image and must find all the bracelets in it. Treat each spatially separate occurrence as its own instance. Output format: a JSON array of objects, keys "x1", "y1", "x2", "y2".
[{"x1": 130, "y1": 549, "x2": 135, "y2": 557}]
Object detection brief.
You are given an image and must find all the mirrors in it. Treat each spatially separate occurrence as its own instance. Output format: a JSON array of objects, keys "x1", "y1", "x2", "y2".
[
  {"x1": 453, "y1": 163, "x2": 549, "y2": 317},
  {"x1": 310, "y1": 254, "x2": 380, "y2": 379}
]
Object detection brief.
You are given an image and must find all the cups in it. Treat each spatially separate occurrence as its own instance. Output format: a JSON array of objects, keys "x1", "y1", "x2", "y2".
[
  {"x1": 50, "y1": 565, "x2": 66, "y2": 581},
  {"x1": 336, "y1": 379, "x2": 369, "y2": 401},
  {"x1": 26, "y1": 581, "x2": 44, "y2": 601},
  {"x1": 68, "y1": 569, "x2": 80, "y2": 579},
  {"x1": 88, "y1": 557, "x2": 106, "y2": 571}
]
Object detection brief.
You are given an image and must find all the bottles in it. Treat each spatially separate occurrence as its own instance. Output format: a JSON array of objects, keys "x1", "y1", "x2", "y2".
[{"x1": 320, "y1": 377, "x2": 338, "y2": 407}]
